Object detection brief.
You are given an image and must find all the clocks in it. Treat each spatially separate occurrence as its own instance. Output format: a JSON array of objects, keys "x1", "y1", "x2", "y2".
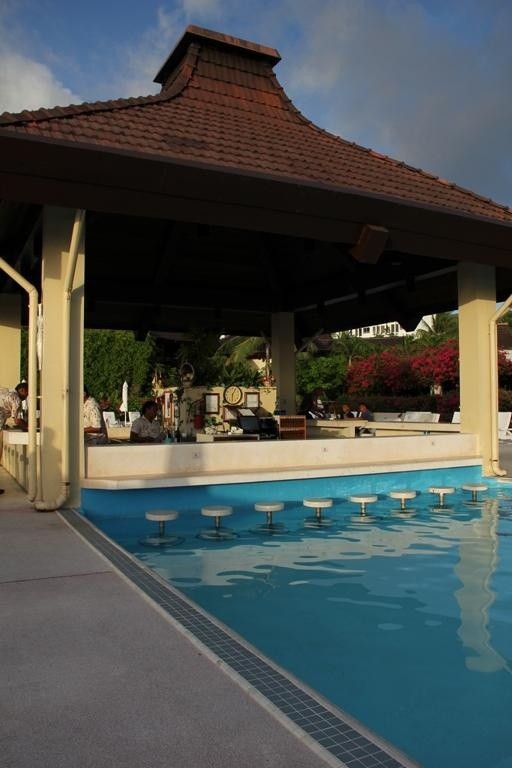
[{"x1": 224, "y1": 386, "x2": 242, "y2": 404}]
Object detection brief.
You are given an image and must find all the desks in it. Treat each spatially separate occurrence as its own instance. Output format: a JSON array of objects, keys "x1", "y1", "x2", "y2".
[{"x1": 196, "y1": 433, "x2": 259, "y2": 442}]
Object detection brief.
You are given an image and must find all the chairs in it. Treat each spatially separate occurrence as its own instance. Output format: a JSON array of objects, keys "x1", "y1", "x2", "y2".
[
  {"x1": 102, "y1": 412, "x2": 123, "y2": 428},
  {"x1": 126, "y1": 412, "x2": 141, "y2": 427}
]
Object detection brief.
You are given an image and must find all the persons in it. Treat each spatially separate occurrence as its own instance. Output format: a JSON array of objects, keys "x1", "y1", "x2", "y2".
[
  {"x1": 129, "y1": 400, "x2": 164, "y2": 442},
  {"x1": 0, "y1": 383, "x2": 28, "y2": 494},
  {"x1": 84, "y1": 384, "x2": 109, "y2": 444},
  {"x1": 356, "y1": 402, "x2": 375, "y2": 421},
  {"x1": 338, "y1": 403, "x2": 354, "y2": 418}
]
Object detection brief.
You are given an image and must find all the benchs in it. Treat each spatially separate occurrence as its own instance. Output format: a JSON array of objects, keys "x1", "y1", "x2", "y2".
[
  {"x1": 324, "y1": 411, "x2": 440, "y2": 433},
  {"x1": 451, "y1": 411, "x2": 512, "y2": 440}
]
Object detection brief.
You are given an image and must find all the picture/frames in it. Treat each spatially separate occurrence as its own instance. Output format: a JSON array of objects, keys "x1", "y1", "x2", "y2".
[
  {"x1": 164, "y1": 391, "x2": 172, "y2": 422},
  {"x1": 203, "y1": 393, "x2": 220, "y2": 414},
  {"x1": 245, "y1": 392, "x2": 260, "y2": 407}
]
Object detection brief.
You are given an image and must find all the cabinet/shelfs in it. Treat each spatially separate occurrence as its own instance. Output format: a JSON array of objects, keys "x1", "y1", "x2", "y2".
[{"x1": 279, "y1": 416, "x2": 307, "y2": 440}]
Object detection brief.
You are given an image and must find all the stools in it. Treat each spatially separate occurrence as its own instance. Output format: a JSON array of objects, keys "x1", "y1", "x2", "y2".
[
  {"x1": 350, "y1": 495, "x2": 378, "y2": 517},
  {"x1": 428, "y1": 487, "x2": 456, "y2": 508},
  {"x1": 254, "y1": 501, "x2": 285, "y2": 527},
  {"x1": 144, "y1": 510, "x2": 178, "y2": 538},
  {"x1": 462, "y1": 483, "x2": 489, "y2": 502},
  {"x1": 201, "y1": 505, "x2": 233, "y2": 530},
  {"x1": 390, "y1": 490, "x2": 417, "y2": 510},
  {"x1": 303, "y1": 498, "x2": 333, "y2": 518}
]
